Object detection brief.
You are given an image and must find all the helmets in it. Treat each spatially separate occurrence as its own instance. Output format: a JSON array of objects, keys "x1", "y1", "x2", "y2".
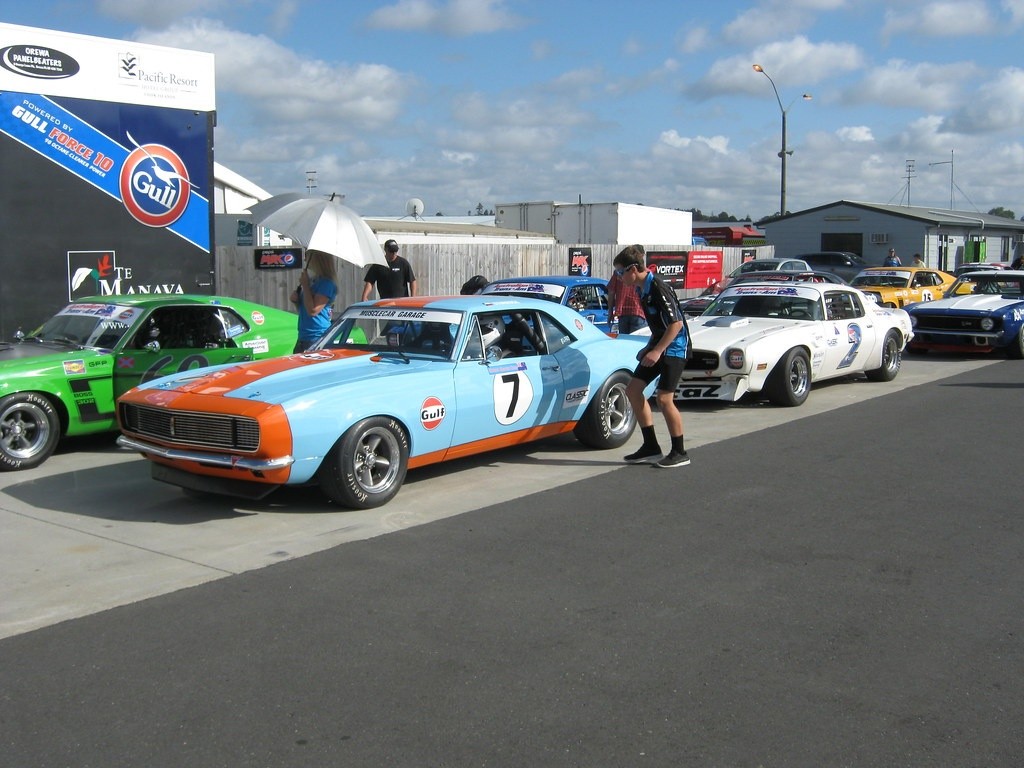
[{"x1": 468, "y1": 315, "x2": 505, "y2": 350}]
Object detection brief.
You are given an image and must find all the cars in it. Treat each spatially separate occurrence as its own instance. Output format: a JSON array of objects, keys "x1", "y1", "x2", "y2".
[
  {"x1": 627, "y1": 281, "x2": 915, "y2": 407},
  {"x1": 385, "y1": 276, "x2": 622, "y2": 351},
  {"x1": 0, "y1": 294, "x2": 367, "y2": 471},
  {"x1": 712, "y1": 258, "x2": 816, "y2": 294},
  {"x1": 952, "y1": 262, "x2": 1020, "y2": 288},
  {"x1": 117, "y1": 294, "x2": 660, "y2": 509},
  {"x1": 847, "y1": 267, "x2": 978, "y2": 309},
  {"x1": 794, "y1": 251, "x2": 885, "y2": 283},
  {"x1": 901, "y1": 270, "x2": 1024, "y2": 359},
  {"x1": 676, "y1": 270, "x2": 851, "y2": 317}
]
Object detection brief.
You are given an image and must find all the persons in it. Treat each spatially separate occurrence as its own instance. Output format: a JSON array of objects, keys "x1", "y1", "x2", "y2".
[
  {"x1": 884, "y1": 248, "x2": 901, "y2": 266},
  {"x1": 475, "y1": 315, "x2": 515, "y2": 357},
  {"x1": 290, "y1": 248, "x2": 337, "y2": 352},
  {"x1": 568, "y1": 291, "x2": 584, "y2": 310},
  {"x1": 607, "y1": 244, "x2": 648, "y2": 334},
  {"x1": 911, "y1": 253, "x2": 925, "y2": 267},
  {"x1": 362, "y1": 238, "x2": 416, "y2": 301},
  {"x1": 613, "y1": 245, "x2": 692, "y2": 468},
  {"x1": 1011, "y1": 255, "x2": 1024, "y2": 270}
]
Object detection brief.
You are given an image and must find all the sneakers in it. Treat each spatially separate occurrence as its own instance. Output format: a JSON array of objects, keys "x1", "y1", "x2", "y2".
[
  {"x1": 624, "y1": 444, "x2": 661, "y2": 463},
  {"x1": 656, "y1": 450, "x2": 690, "y2": 467}
]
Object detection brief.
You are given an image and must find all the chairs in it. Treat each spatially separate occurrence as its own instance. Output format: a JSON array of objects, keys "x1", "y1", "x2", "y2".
[
  {"x1": 917, "y1": 276, "x2": 936, "y2": 286},
  {"x1": 410, "y1": 322, "x2": 453, "y2": 357},
  {"x1": 501, "y1": 322, "x2": 538, "y2": 356},
  {"x1": 731, "y1": 293, "x2": 783, "y2": 318}
]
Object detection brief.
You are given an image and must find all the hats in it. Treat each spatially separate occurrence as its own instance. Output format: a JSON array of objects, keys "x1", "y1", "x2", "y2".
[
  {"x1": 889, "y1": 248, "x2": 895, "y2": 252},
  {"x1": 385, "y1": 239, "x2": 398, "y2": 250}
]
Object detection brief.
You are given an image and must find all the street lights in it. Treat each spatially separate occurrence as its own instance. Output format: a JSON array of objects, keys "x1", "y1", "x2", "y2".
[{"x1": 753, "y1": 65, "x2": 813, "y2": 216}]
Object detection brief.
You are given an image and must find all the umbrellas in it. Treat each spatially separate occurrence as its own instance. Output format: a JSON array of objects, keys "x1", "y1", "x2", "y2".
[{"x1": 244, "y1": 192, "x2": 390, "y2": 294}]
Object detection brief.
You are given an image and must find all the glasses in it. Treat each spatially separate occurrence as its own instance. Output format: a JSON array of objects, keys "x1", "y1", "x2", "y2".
[{"x1": 617, "y1": 264, "x2": 639, "y2": 275}]
